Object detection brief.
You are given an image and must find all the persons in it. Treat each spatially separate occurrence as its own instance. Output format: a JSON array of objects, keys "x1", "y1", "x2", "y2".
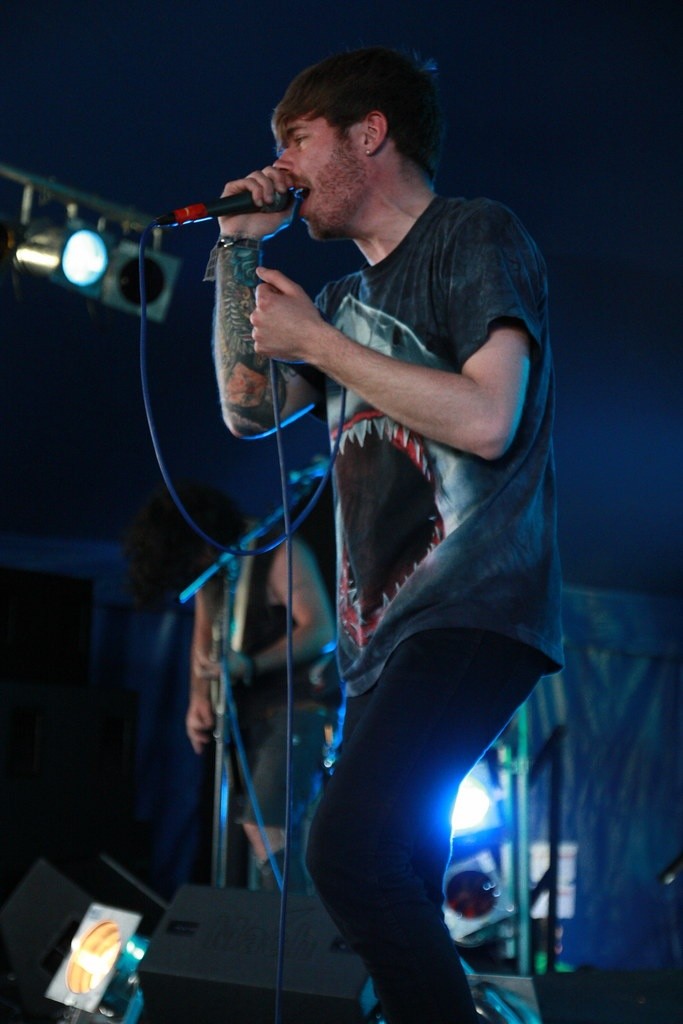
[
  {"x1": 213, "y1": 46, "x2": 561, "y2": 1024},
  {"x1": 131, "y1": 488, "x2": 347, "y2": 899}
]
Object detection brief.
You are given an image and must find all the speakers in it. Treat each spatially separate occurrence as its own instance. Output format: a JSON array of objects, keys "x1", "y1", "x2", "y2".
[
  {"x1": 135, "y1": 882, "x2": 380, "y2": 1024},
  {"x1": 0, "y1": 856, "x2": 95, "y2": 1017}
]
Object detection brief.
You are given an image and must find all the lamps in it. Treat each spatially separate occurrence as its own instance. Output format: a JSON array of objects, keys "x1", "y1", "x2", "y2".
[
  {"x1": 450, "y1": 766, "x2": 502, "y2": 836},
  {"x1": 100, "y1": 239, "x2": 182, "y2": 324},
  {"x1": 44, "y1": 900, "x2": 154, "y2": 1020},
  {"x1": 440, "y1": 850, "x2": 516, "y2": 939},
  {"x1": 11, "y1": 224, "x2": 107, "y2": 285}
]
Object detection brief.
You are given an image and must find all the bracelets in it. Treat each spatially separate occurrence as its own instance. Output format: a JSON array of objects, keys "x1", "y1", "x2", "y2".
[
  {"x1": 216, "y1": 233, "x2": 262, "y2": 252},
  {"x1": 240, "y1": 653, "x2": 256, "y2": 686}
]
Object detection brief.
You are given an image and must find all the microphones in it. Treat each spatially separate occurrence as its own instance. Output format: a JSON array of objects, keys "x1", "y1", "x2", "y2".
[
  {"x1": 157, "y1": 183, "x2": 293, "y2": 226},
  {"x1": 285, "y1": 459, "x2": 330, "y2": 484}
]
